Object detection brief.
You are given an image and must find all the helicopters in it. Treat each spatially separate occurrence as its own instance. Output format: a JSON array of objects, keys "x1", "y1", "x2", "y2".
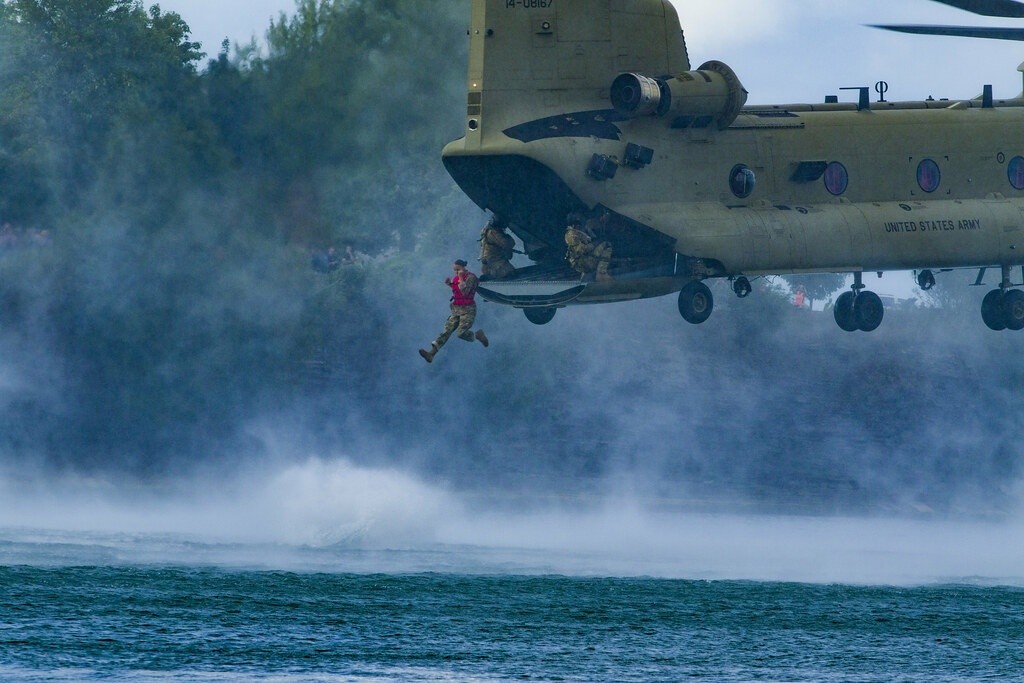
[{"x1": 442, "y1": 0, "x2": 1024, "y2": 331}]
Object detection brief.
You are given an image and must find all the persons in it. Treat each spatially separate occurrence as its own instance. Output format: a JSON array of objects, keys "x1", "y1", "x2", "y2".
[
  {"x1": 418, "y1": 260, "x2": 489, "y2": 363},
  {"x1": 476, "y1": 212, "x2": 515, "y2": 280},
  {"x1": 564, "y1": 210, "x2": 614, "y2": 282}
]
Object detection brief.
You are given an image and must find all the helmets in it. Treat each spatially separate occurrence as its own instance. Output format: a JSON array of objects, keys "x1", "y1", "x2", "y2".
[
  {"x1": 567, "y1": 212, "x2": 584, "y2": 225},
  {"x1": 491, "y1": 212, "x2": 508, "y2": 228}
]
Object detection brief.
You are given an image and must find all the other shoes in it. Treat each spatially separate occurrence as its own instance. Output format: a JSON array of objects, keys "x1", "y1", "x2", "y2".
[
  {"x1": 478, "y1": 273, "x2": 491, "y2": 281},
  {"x1": 474, "y1": 329, "x2": 489, "y2": 348},
  {"x1": 418, "y1": 348, "x2": 434, "y2": 363},
  {"x1": 595, "y1": 273, "x2": 613, "y2": 281}
]
